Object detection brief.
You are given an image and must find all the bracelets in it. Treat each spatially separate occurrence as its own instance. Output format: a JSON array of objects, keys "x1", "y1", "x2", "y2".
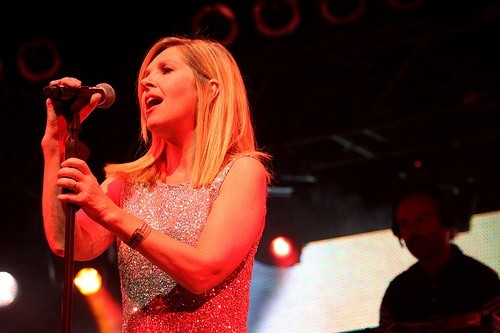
[{"x1": 122, "y1": 220, "x2": 153, "y2": 250}]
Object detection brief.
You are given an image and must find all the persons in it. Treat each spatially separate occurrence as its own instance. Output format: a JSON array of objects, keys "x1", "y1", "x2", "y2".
[
  {"x1": 379, "y1": 173, "x2": 500, "y2": 333},
  {"x1": 41, "y1": 36, "x2": 278, "y2": 333}
]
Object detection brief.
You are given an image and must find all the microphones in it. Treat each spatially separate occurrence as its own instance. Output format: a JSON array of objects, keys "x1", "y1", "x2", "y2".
[{"x1": 44, "y1": 82, "x2": 115, "y2": 110}]
